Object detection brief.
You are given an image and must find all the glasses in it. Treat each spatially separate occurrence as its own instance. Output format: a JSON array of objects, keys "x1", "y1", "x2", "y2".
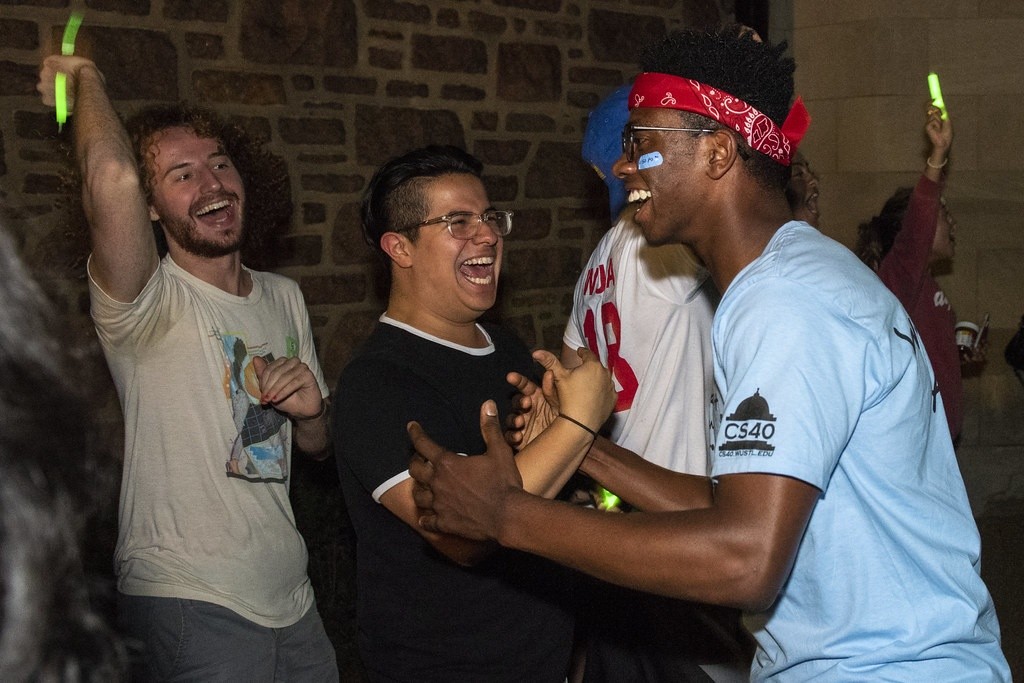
[
  {"x1": 396, "y1": 210, "x2": 514, "y2": 239},
  {"x1": 620, "y1": 124, "x2": 749, "y2": 162},
  {"x1": 938, "y1": 211, "x2": 955, "y2": 226}
]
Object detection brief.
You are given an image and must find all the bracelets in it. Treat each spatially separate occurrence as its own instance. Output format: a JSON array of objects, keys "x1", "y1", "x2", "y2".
[
  {"x1": 926, "y1": 156, "x2": 948, "y2": 169},
  {"x1": 559, "y1": 414, "x2": 597, "y2": 438}
]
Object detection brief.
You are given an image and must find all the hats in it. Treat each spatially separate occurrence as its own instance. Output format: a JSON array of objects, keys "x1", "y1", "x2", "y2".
[{"x1": 580, "y1": 83, "x2": 633, "y2": 219}]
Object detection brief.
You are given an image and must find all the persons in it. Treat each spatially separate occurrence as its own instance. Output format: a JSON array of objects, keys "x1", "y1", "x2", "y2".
[
  {"x1": 340, "y1": 144, "x2": 615, "y2": 683},
  {"x1": 406, "y1": 26, "x2": 1013, "y2": 683},
  {"x1": 36, "y1": 54, "x2": 345, "y2": 683}
]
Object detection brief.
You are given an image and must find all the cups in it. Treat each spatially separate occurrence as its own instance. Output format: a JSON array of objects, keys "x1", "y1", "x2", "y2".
[{"x1": 954, "y1": 321, "x2": 979, "y2": 365}]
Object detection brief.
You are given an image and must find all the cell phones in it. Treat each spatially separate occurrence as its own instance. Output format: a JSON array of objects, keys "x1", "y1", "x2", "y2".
[{"x1": 974, "y1": 312, "x2": 991, "y2": 351}]
